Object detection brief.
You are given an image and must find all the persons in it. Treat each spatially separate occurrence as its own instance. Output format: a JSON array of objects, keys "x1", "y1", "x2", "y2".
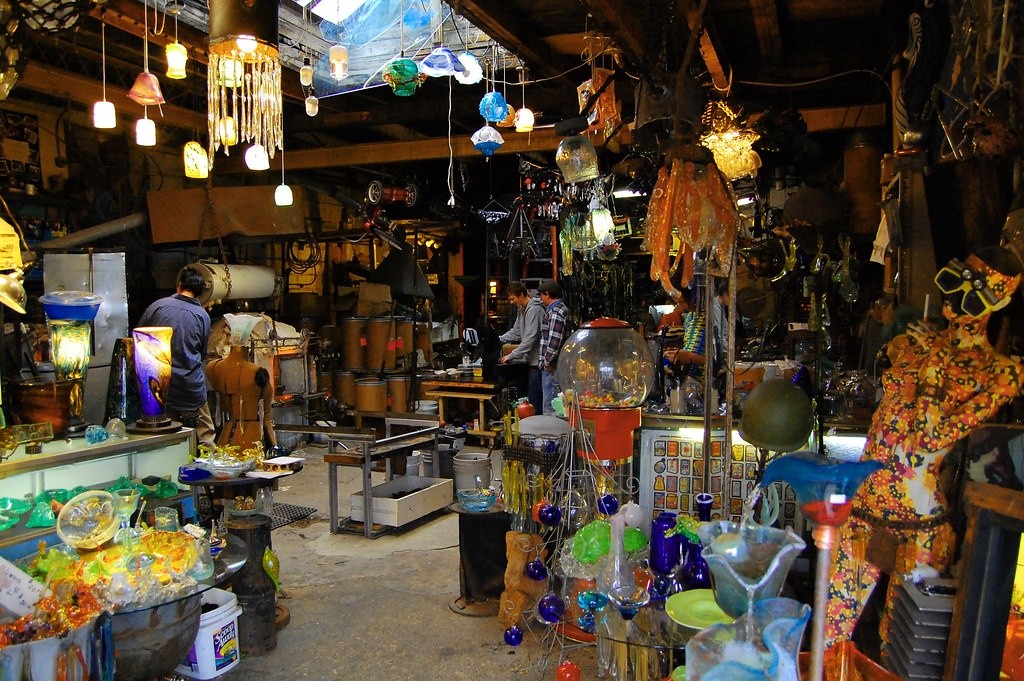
[
  {"x1": 663, "y1": 280, "x2": 729, "y2": 417},
  {"x1": 824, "y1": 248, "x2": 1024, "y2": 669},
  {"x1": 137, "y1": 267, "x2": 216, "y2": 443},
  {"x1": 206, "y1": 346, "x2": 277, "y2": 455},
  {"x1": 498, "y1": 281, "x2": 546, "y2": 415},
  {"x1": 536, "y1": 280, "x2": 572, "y2": 416}
]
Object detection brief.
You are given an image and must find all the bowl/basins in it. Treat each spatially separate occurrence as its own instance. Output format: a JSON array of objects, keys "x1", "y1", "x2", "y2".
[{"x1": 436, "y1": 364, "x2": 482, "y2": 377}]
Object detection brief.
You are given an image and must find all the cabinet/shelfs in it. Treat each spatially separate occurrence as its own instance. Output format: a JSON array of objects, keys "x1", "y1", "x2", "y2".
[{"x1": 0, "y1": 427, "x2": 199, "y2": 562}]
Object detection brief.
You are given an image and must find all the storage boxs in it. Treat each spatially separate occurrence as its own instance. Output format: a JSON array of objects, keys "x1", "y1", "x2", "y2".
[{"x1": 351, "y1": 475, "x2": 454, "y2": 528}]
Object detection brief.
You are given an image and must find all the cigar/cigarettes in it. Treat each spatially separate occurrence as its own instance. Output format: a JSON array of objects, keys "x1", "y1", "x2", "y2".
[{"x1": 923, "y1": 294, "x2": 929, "y2": 322}]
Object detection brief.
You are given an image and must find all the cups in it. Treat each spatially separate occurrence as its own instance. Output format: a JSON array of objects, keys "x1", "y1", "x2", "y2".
[
  {"x1": 54, "y1": 489, "x2": 214, "y2": 604},
  {"x1": 26, "y1": 184, "x2": 37, "y2": 195}
]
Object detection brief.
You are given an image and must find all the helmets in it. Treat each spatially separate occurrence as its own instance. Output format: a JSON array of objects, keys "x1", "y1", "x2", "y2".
[{"x1": 739, "y1": 377, "x2": 815, "y2": 451}]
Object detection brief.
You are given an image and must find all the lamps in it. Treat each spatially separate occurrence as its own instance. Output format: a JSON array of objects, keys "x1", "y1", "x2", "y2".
[
  {"x1": 184, "y1": 61, "x2": 209, "y2": 178},
  {"x1": 330, "y1": 0, "x2": 348, "y2": 80},
  {"x1": 554, "y1": 116, "x2": 599, "y2": 184},
  {"x1": 453, "y1": 1, "x2": 483, "y2": 85},
  {"x1": 94, "y1": 0, "x2": 187, "y2": 146},
  {"x1": 0, "y1": 261, "x2": 36, "y2": 314},
  {"x1": 127, "y1": 326, "x2": 183, "y2": 434},
  {"x1": 39, "y1": 293, "x2": 103, "y2": 439},
  {"x1": 699, "y1": 97, "x2": 762, "y2": 181},
  {"x1": 381, "y1": 0, "x2": 418, "y2": 97},
  {"x1": 415, "y1": 0, "x2": 466, "y2": 78},
  {"x1": 207, "y1": 0, "x2": 293, "y2": 207},
  {"x1": 471, "y1": 40, "x2": 535, "y2": 159}
]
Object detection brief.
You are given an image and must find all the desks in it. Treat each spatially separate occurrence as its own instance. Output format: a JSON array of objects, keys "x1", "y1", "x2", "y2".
[
  {"x1": 17, "y1": 525, "x2": 249, "y2": 681},
  {"x1": 550, "y1": 576, "x2": 698, "y2": 681},
  {"x1": 179, "y1": 464, "x2": 304, "y2": 519}
]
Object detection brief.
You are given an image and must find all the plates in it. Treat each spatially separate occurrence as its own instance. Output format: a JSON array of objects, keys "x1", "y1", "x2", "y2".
[{"x1": 665, "y1": 588, "x2": 735, "y2": 631}]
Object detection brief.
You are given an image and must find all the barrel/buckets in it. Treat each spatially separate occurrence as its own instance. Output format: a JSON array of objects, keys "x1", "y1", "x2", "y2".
[
  {"x1": 313, "y1": 420, "x2": 337, "y2": 443},
  {"x1": 290, "y1": 293, "x2": 443, "y2": 431},
  {"x1": 386, "y1": 435, "x2": 494, "y2": 496}
]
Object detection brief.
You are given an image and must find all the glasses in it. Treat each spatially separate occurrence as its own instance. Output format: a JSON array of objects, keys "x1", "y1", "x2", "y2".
[{"x1": 934, "y1": 266, "x2": 993, "y2": 320}]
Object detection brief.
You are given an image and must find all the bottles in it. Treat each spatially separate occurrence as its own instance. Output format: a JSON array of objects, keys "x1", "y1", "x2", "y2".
[
  {"x1": 235, "y1": 486, "x2": 273, "y2": 517},
  {"x1": 597, "y1": 493, "x2": 715, "y2": 600}
]
